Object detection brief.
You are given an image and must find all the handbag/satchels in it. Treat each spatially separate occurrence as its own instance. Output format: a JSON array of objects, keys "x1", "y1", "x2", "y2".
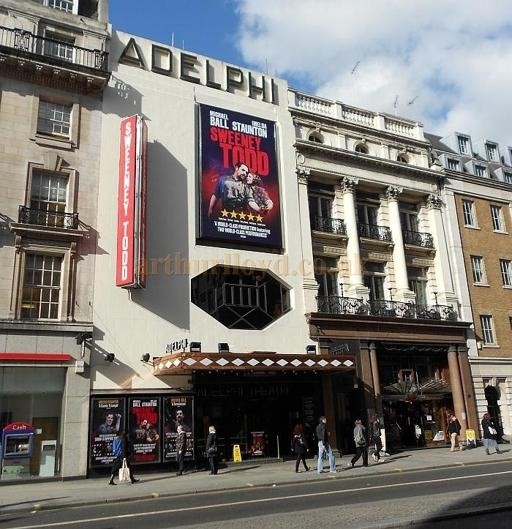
[
  {"x1": 299, "y1": 436, "x2": 306, "y2": 445},
  {"x1": 118, "y1": 457, "x2": 133, "y2": 484},
  {"x1": 486, "y1": 419, "x2": 498, "y2": 435},
  {"x1": 317, "y1": 423, "x2": 331, "y2": 441},
  {"x1": 208, "y1": 438, "x2": 217, "y2": 454},
  {"x1": 353, "y1": 427, "x2": 366, "y2": 445}
]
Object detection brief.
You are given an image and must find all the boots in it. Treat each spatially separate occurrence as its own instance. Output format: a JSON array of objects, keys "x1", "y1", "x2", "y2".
[
  {"x1": 130, "y1": 470, "x2": 139, "y2": 484},
  {"x1": 496, "y1": 448, "x2": 502, "y2": 454},
  {"x1": 485, "y1": 448, "x2": 491, "y2": 455},
  {"x1": 108, "y1": 473, "x2": 117, "y2": 485}
]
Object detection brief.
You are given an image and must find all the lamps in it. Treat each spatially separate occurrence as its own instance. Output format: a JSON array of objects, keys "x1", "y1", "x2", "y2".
[
  {"x1": 304, "y1": 344, "x2": 317, "y2": 355},
  {"x1": 140, "y1": 352, "x2": 155, "y2": 367},
  {"x1": 188, "y1": 341, "x2": 203, "y2": 352},
  {"x1": 217, "y1": 341, "x2": 230, "y2": 353}
]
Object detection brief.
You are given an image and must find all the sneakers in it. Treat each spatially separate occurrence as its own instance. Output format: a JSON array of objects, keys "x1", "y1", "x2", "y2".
[
  {"x1": 377, "y1": 458, "x2": 384, "y2": 464},
  {"x1": 371, "y1": 453, "x2": 377, "y2": 462}
]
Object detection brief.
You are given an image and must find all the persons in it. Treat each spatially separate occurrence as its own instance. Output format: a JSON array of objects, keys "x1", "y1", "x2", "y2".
[
  {"x1": 368, "y1": 414, "x2": 383, "y2": 463},
  {"x1": 289, "y1": 424, "x2": 310, "y2": 473},
  {"x1": 165, "y1": 404, "x2": 191, "y2": 433},
  {"x1": 144, "y1": 422, "x2": 159, "y2": 442},
  {"x1": 132, "y1": 418, "x2": 147, "y2": 440},
  {"x1": 446, "y1": 415, "x2": 462, "y2": 451},
  {"x1": 205, "y1": 426, "x2": 221, "y2": 475},
  {"x1": 246, "y1": 173, "x2": 272, "y2": 214},
  {"x1": 315, "y1": 416, "x2": 338, "y2": 473},
  {"x1": 349, "y1": 419, "x2": 369, "y2": 467},
  {"x1": 98, "y1": 412, "x2": 121, "y2": 433},
  {"x1": 107, "y1": 430, "x2": 138, "y2": 485},
  {"x1": 481, "y1": 413, "x2": 501, "y2": 455},
  {"x1": 174, "y1": 423, "x2": 187, "y2": 476},
  {"x1": 207, "y1": 158, "x2": 264, "y2": 221}
]
